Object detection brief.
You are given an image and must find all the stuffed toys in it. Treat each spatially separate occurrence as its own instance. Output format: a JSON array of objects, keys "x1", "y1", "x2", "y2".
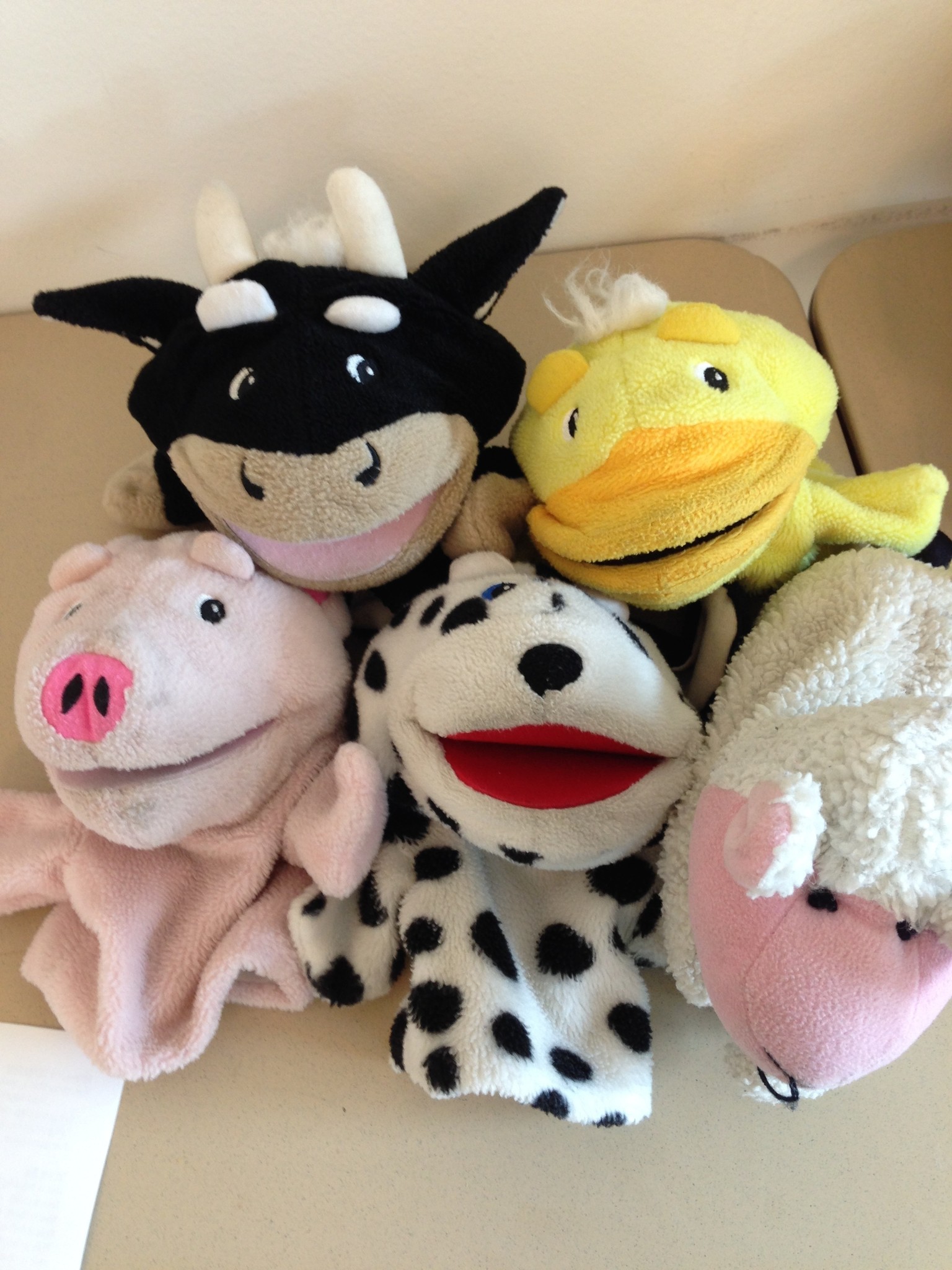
[
  {"x1": 289, "y1": 542, "x2": 709, "y2": 1133},
  {"x1": 508, "y1": 248, "x2": 949, "y2": 611},
  {"x1": 650, "y1": 544, "x2": 949, "y2": 1105},
  {"x1": 36, "y1": 157, "x2": 562, "y2": 618},
  {"x1": 0, "y1": 523, "x2": 384, "y2": 1074}
]
